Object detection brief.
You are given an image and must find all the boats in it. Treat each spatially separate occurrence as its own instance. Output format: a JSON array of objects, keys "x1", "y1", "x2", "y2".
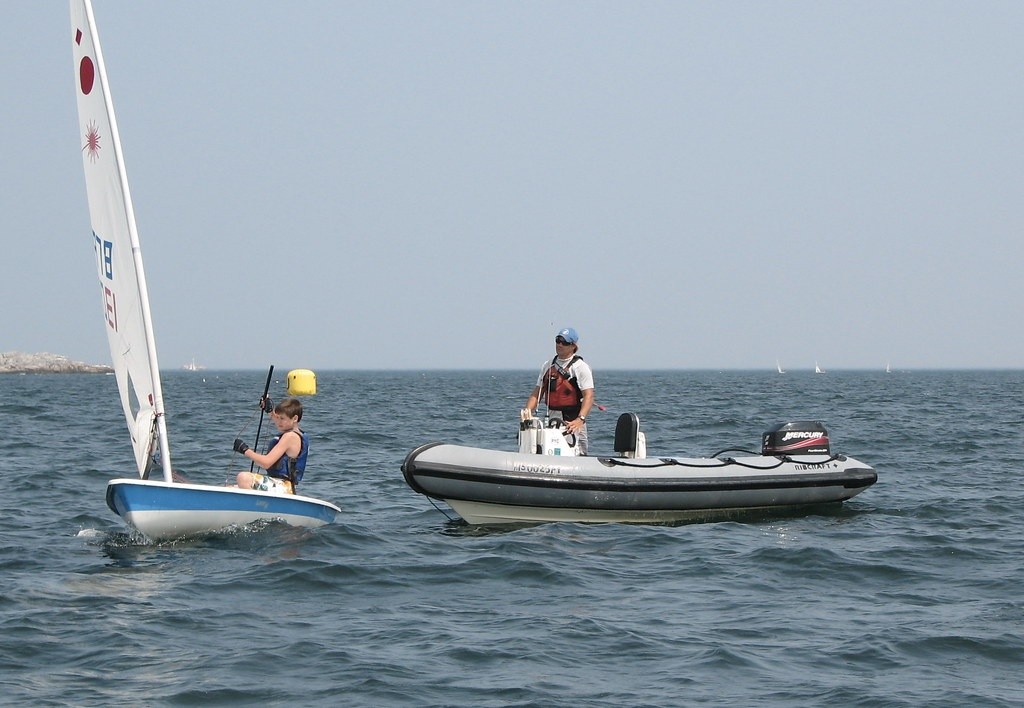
[{"x1": 398, "y1": 365, "x2": 878, "y2": 528}]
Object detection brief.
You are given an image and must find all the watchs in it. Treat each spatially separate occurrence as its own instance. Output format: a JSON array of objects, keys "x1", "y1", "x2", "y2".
[{"x1": 578, "y1": 415, "x2": 585, "y2": 423}]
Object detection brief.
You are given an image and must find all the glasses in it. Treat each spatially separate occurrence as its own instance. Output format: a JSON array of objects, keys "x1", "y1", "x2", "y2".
[{"x1": 556, "y1": 338, "x2": 573, "y2": 346}]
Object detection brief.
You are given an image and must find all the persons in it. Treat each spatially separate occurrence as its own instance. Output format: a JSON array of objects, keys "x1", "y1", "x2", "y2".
[
  {"x1": 234, "y1": 399, "x2": 310, "y2": 495},
  {"x1": 526, "y1": 328, "x2": 594, "y2": 455}
]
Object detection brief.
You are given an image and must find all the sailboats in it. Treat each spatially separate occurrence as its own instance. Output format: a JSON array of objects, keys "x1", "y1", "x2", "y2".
[{"x1": 67, "y1": 0, "x2": 343, "y2": 546}]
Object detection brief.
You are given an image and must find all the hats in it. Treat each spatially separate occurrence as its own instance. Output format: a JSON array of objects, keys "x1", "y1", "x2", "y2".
[{"x1": 555, "y1": 328, "x2": 578, "y2": 343}]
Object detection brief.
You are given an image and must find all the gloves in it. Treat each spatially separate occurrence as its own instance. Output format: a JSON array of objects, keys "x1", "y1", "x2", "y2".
[{"x1": 233, "y1": 439, "x2": 249, "y2": 454}]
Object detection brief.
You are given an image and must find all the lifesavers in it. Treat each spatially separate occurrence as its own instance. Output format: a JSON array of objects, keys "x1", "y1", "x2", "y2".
[{"x1": 624, "y1": 431, "x2": 646, "y2": 459}]
